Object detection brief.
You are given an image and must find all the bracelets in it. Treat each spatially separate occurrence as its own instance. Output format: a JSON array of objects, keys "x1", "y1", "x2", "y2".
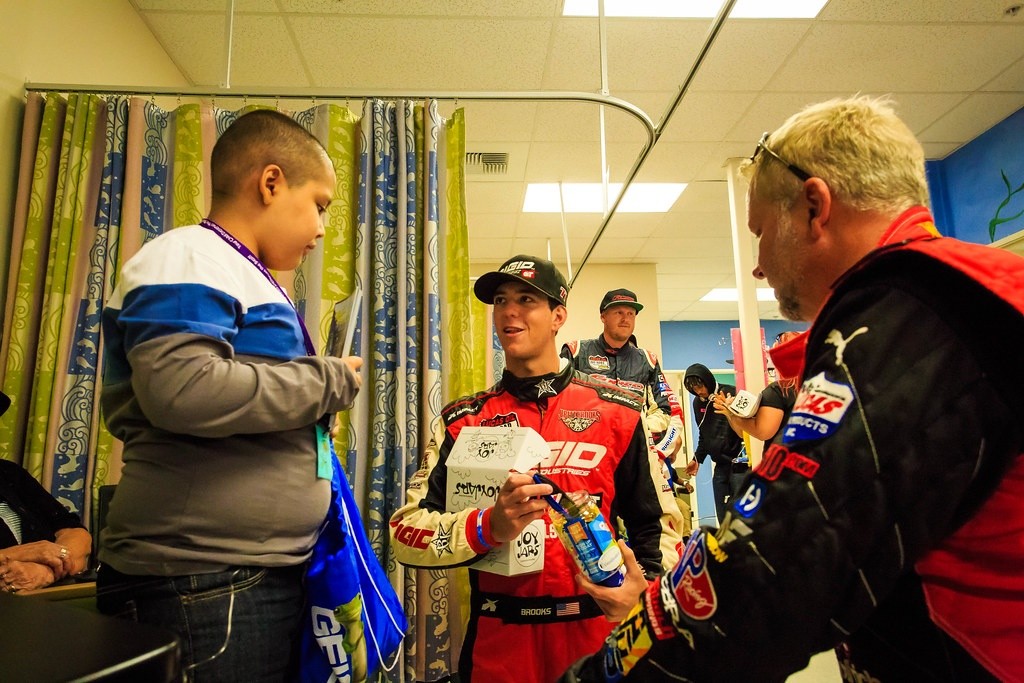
[
  {"x1": 478, "y1": 506, "x2": 497, "y2": 549},
  {"x1": 683, "y1": 480, "x2": 689, "y2": 486}
]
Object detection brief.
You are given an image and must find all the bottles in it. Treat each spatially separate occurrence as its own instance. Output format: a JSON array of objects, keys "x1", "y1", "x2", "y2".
[{"x1": 547, "y1": 489, "x2": 628, "y2": 588}]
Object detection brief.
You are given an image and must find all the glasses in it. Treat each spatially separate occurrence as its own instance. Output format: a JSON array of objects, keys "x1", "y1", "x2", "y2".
[
  {"x1": 775, "y1": 332, "x2": 785, "y2": 345},
  {"x1": 750, "y1": 132, "x2": 811, "y2": 182}
]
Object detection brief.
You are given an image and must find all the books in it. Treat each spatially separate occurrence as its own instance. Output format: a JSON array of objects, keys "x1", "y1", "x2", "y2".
[{"x1": 320, "y1": 287, "x2": 362, "y2": 430}]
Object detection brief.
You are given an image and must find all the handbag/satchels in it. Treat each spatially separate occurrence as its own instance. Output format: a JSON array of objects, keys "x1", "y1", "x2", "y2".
[{"x1": 301, "y1": 440, "x2": 409, "y2": 683}]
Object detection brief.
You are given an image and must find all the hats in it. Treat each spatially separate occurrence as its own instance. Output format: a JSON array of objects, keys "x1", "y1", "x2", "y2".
[
  {"x1": 600, "y1": 288, "x2": 644, "y2": 314},
  {"x1": 474, "y1": 255, "x2": 568, "y2": 307}
]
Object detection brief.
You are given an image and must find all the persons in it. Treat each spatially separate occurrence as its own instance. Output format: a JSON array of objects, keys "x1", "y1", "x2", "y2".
[
  {"x1": 99, "y1": 105, "x2": 363, "y2": 682},
  {"x1": 388, "y1": 253, "x2": 686, "y2": 682},
  {"x1": 0, "y1": 388, "x2": 94, "y2": 596},
  {"x1": 562, "y1": 283, "x2": 686, "y2": 587},
  {"x1": 682, "y1": 361, "x2": 747, "y2": 531},
  {"x1": 714, "y1": 330, "x2": 807, "y2": 468},
  {"x1": 556, "y1": 96, "x2": 1024, "y2": 682},
  {"x1": 660, "y1": 434, "x2": 697, "y2": 536}
]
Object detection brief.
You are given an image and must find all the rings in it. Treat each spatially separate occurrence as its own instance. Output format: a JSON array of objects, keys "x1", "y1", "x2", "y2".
[
  {"x1": 1, "y1": 573, "x2": 8, "y2": 583},
  {"x1": 60, "y1": 547, "x2": 67, "y2": 558},
  {"x1": 6, "y1": 584, "x2": 18, "y2": 593}
]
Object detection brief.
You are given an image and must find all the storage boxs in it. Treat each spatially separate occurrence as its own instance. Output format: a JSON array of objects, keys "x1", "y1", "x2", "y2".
[{"x1": 444, "y1": 427, "x2": 551, "y2": 576}]
